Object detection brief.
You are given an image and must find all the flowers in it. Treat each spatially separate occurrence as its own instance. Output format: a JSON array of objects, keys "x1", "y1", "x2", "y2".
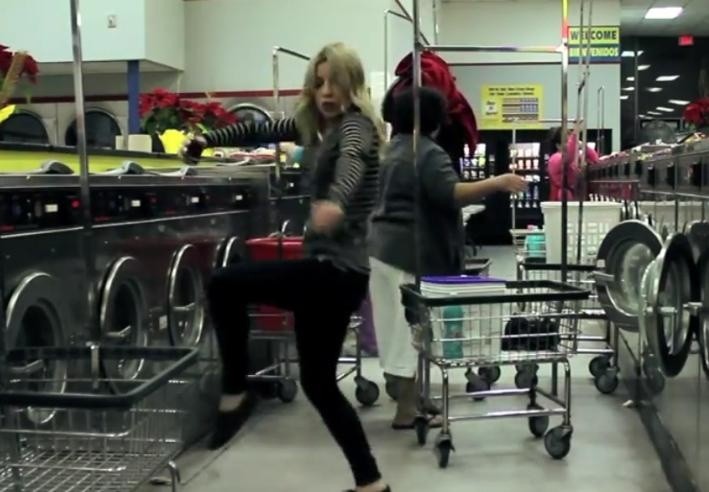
[
  {"x1": 139, "y1": 89, "x2": 238, "y2": 137},
  {"x1": 684, "y1": 95, "x2": 709, "y2": 130},
  {"x1": 0, "y1": 45, "x2": 38, "y2": 122}
]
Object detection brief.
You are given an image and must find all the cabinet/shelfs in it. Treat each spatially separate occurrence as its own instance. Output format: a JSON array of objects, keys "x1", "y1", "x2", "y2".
[{"x1": 459, "y1": 129, "x2": 614, "y2": 243}]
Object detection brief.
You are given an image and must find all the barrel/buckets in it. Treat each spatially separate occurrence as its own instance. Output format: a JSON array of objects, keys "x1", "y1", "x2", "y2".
[
  {"x1": 523, "y1": 230, "x2": 545, "y2": 259},
  {"x1": 420, "y1": 277, "x2": 507, "y2": 361}
]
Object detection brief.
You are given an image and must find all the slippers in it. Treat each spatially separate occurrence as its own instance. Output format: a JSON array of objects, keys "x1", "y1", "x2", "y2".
[{"x1": 391, "y1": 413, "x2": 451, "y2": 430}]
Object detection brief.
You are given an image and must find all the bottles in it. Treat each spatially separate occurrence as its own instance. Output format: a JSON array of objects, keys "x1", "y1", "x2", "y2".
[
  {"x1": 460, "y1": 142, "x2": 488, "y2": 181},
  {"x1": 444, "y1": 290, "x2": 465, "y2": 360},
  {"x1": 509, "y1": 146, "x2": 540, "y2": 208},
  {"x1": 526, "y1": 224, "x2": 546, "y2": 258}
]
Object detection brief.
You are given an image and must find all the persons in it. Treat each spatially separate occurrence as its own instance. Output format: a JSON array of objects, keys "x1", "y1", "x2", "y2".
[
  {"x1": 181, "y1": 44, "x2": 392, "y2": 492},
  {"x1": 548, "y1": 127, "x2": 598, "y2": 201},
  {"x1": 368, "y1": 87, "x2": 526, "y2": 428}
]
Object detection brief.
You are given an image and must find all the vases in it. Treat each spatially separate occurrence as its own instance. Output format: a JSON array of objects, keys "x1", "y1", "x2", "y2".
[{"x1": 157, "y1": 127, "x2": 190, "y2": 154}]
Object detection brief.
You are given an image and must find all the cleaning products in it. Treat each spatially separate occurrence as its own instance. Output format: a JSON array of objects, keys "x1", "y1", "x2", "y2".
[{"x1": 443, "y1": 293, "x2": 464, "y2": 357}]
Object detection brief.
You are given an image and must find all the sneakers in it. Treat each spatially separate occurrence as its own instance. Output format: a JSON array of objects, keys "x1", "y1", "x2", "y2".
[{"x1": 204, "y1": 386, "x2": 262, "y2": 453}]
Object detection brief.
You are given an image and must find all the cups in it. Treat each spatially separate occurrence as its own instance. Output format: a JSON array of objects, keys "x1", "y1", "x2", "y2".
[{"x1": 182, "y1": 140, "x2": 206, "y2": 166}]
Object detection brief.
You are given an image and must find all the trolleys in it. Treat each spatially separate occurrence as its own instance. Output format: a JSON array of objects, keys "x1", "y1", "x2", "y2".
[{"x1": 2, "y1": 2, "x2": 210, "y2": 492}]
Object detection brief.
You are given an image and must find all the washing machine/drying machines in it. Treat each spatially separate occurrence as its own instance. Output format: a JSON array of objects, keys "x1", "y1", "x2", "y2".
[
  {"x1": 587, "y1": 138, "x2": 709, "y2": 492},
  {"x1": 0, "y1": 161, "x2": 319, "y2": 492}
]
